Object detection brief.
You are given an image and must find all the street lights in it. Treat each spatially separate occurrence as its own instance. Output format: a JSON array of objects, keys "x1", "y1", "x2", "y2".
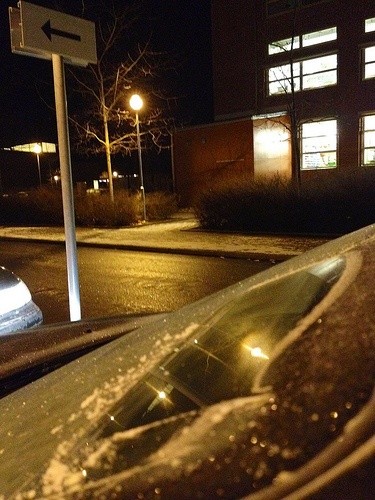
[{"x1": 129, "y1": 94, "x2": 148, "y2": 220}]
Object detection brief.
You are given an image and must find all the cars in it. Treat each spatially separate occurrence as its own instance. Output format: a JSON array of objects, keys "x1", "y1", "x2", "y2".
[{"x1": 0, "y1": 221, "x2": 374, "y2": 494}]
